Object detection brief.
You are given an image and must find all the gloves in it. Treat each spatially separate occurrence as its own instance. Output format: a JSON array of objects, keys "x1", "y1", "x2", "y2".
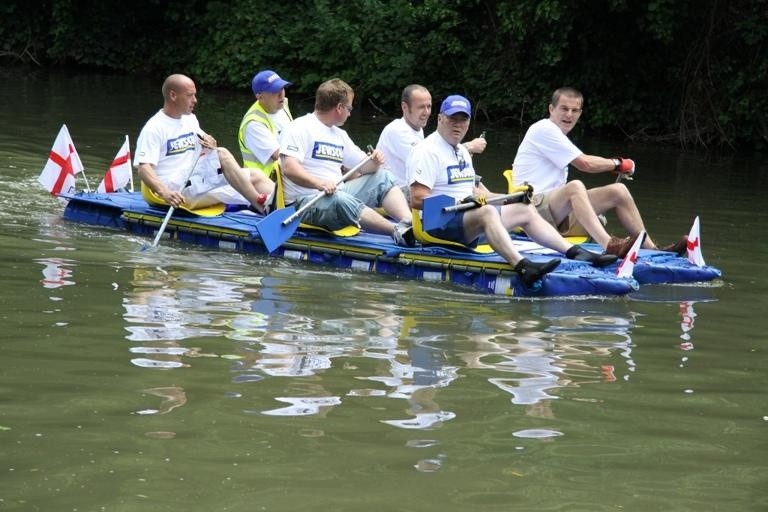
[{"x1": 612, "y1": 156, "x2": 636, "y2": 180}]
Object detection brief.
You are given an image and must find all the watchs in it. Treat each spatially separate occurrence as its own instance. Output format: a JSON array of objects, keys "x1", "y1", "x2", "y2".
[{"x1": 613, "y1": 158, "x2": 620, "y2": 171}]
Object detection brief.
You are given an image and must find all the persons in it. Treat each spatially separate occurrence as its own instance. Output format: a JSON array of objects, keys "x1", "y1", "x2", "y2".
[
  {"x1": 132, "y1": 72, "x2": 276, "y2": 215},
  {"x1": 237, "y1": 69, "x2": 294, "y2": 183},
  {"x1": 405, "y1": 95, "x2": 619, "y2": 289},
  {"x1": 278, "y1": 77, "x2": 415, "y2": 248},
  {"x1": 374, "y1": 83, "x2": 492, "y2": 213},
  {"x1": 510, "y1": 85, "x2": 690, "y2": 260}
]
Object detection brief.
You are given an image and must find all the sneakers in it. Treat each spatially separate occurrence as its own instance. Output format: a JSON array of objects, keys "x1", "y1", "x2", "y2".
[
  {"x1": 566, "y1": 244, "x2": 618, "y2": 267},
  {"x1": 514, "y1": 257, "x2": 562, "y2": 290},
  {"x1": 393, "y1": 223, "x2": 417, "y2": 249},
  {"x1": 257, "y1": 181, "x2": 278, "y2": 218},
  {"x1": 654, "y1": 235, "x2": 689, "y2": 257},
  {"x1": 606, "y1": 231, "x2": 646, "y2": 260}
]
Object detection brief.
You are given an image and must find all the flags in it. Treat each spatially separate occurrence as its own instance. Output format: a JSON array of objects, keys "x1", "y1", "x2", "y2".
[
  {"x1": 37, "y1": 124, "x2": 85, "y2": 202},
  {"x1": 94, "y1": 138, "x2": 132, "y2": 194},
  {"x1": 615, "y1": 228, "x2": 646, "y2": 279},
  {"x1": 686, "y1": 215, "x2": 706, "y2": 269}
]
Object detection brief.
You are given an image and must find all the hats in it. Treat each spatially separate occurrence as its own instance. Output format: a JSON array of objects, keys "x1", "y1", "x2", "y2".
[
  {"x1": 440, "y1": 94, "x2": 472, "y2": 120},
  {"x1": 251, "y1": 70, "x2": 292, "y2": 97}
]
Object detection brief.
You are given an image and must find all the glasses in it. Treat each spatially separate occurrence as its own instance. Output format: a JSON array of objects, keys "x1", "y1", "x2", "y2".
[{"x1": 339, "y1": 102, "x2": 354, "y2": 114}]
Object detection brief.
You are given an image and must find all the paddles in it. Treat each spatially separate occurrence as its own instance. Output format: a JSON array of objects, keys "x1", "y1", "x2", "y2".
[{"x1": 255, "y1": 144, "x2": 375, "y2": 253}]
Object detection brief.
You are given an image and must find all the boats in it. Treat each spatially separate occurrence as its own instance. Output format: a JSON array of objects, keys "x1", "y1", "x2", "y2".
[
  {"x1": 114, "y1": 191, "x2": 723, "y2": 284},
  {"x1": 61, "y1": 189, "x2": 640, "y2": 299}
]
